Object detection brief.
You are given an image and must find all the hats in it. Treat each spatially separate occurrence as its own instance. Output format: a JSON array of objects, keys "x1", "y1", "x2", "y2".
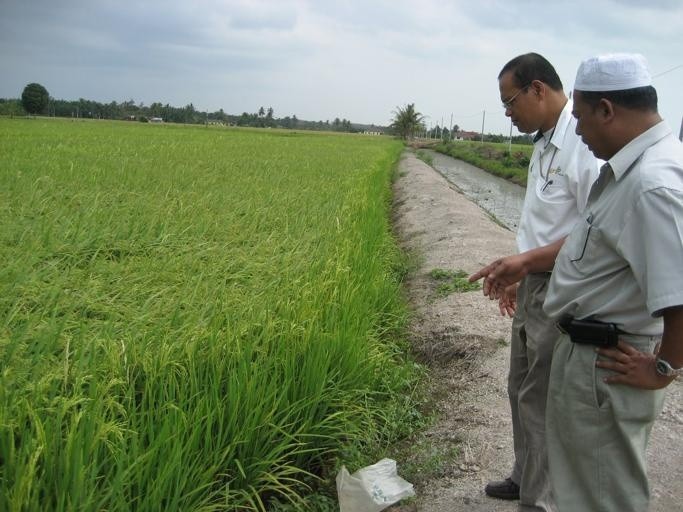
[{"x1": 573, "y1": 51, "x2": 653, "y2": 92}]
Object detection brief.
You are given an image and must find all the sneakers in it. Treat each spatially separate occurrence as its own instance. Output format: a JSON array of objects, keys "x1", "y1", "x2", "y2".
[{"x1": 484, "y1": 480, "x2": 522, "y2": 501}]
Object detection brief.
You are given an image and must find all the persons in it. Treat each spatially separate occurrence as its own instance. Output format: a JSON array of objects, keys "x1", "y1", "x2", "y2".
[
  {"x1": 469, "y1": 49, "x2": 683, "y2": 512},
  {"x1": 484, "y1": 51, "x2": 609, "y2": 501}
]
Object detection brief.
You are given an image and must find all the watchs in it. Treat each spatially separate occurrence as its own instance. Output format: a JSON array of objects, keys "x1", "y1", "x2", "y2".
[{"x1": 653, "y1": 355, "x2": 683, "y2": 378}]
